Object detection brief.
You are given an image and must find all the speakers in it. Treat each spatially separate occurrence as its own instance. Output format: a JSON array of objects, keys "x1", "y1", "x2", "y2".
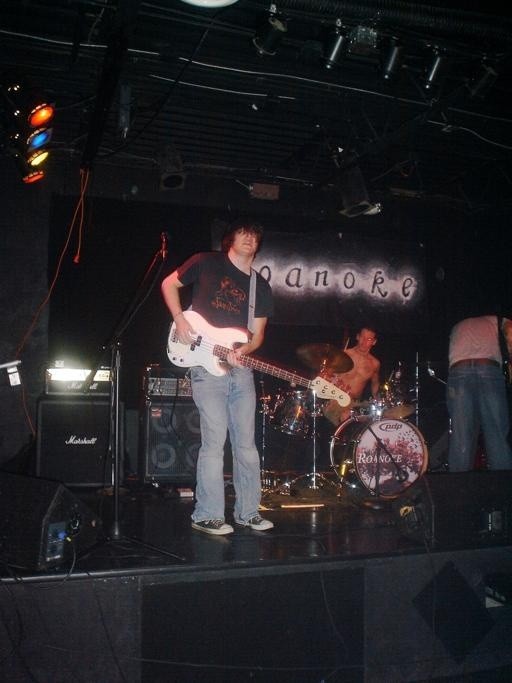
[
  {"x1": 144, "y1": 403, "x2": 201, "y2": 484},
  {"x1": 35, "y1": 393, "x2": 122, "y2": 485}
]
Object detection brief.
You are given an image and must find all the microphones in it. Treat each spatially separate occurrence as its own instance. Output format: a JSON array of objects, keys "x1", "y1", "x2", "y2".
[{"x1": 159, "y1": 231, "x2": 168, "y2": 258}]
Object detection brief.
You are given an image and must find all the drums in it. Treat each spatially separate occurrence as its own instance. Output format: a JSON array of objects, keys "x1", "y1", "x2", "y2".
[
  {"x1": 269, "y1": 389, "x2": 319, "y2": 438},
  {"x1": 349, "y1": 399, "x2": 385, "y2": 421},
  {"x1": 328, "y1": 417, "x2": 430, "y2": 500}
]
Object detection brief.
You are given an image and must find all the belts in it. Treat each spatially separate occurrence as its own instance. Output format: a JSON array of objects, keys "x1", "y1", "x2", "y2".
[{"x1": 453, "y1": 358, "x2": 503, "y2": 370}]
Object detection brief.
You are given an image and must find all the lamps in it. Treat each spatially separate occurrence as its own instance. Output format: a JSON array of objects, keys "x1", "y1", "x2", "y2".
[
  {"x1": 252, "y1": 9, "x2": 287, "y2": 60},
  {"x1": 381, "y1": 33, "x2": 406, "y2": 83},
  {"x1": 422, "y1": 43, "x2": 449, "y2": 89},
  {"x1": 322, "y1": 21, "x2": 348, "y2": 67}
]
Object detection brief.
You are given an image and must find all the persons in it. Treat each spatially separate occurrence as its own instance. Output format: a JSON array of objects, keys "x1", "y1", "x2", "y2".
[
  {"x1": 304, "y1": 326, "x2": 381, "y2": 476},
  {"x1": 447, "y1": 313, "x2": 512, "y2": 471},
  {"x1": 161, "y1": 224, "x2": 275, "y2": 535}
]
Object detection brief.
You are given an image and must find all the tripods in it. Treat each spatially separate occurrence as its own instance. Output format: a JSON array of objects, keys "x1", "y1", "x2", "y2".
[
  {"x1": 48, "y1": 253, "x2": 188, "y2": 575},
  {"x1": 281, "y1": 390, "x2": 339, "y2": 490}
]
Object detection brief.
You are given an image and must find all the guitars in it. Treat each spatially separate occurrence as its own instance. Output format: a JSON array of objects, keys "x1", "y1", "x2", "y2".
[{"x1": 167, "y1": 310, "x2": 352, "y2": 409}]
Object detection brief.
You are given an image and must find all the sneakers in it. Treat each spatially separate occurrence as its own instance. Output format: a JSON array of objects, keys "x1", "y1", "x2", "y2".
[
  {"x1": 235, "y1": 516, "x2": 276, "y2": 531},
  {"x1": 190, "y1": 520, "x2": 235, "y2": 536}
]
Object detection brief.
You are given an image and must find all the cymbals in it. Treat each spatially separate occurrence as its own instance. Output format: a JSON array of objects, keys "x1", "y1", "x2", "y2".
[
  {"x1": 295, "y1": 343, "x2": 355, "y2": 374},
  {"x1": 379, "y1": 378, "x2": 416, "y2": 420}
]
